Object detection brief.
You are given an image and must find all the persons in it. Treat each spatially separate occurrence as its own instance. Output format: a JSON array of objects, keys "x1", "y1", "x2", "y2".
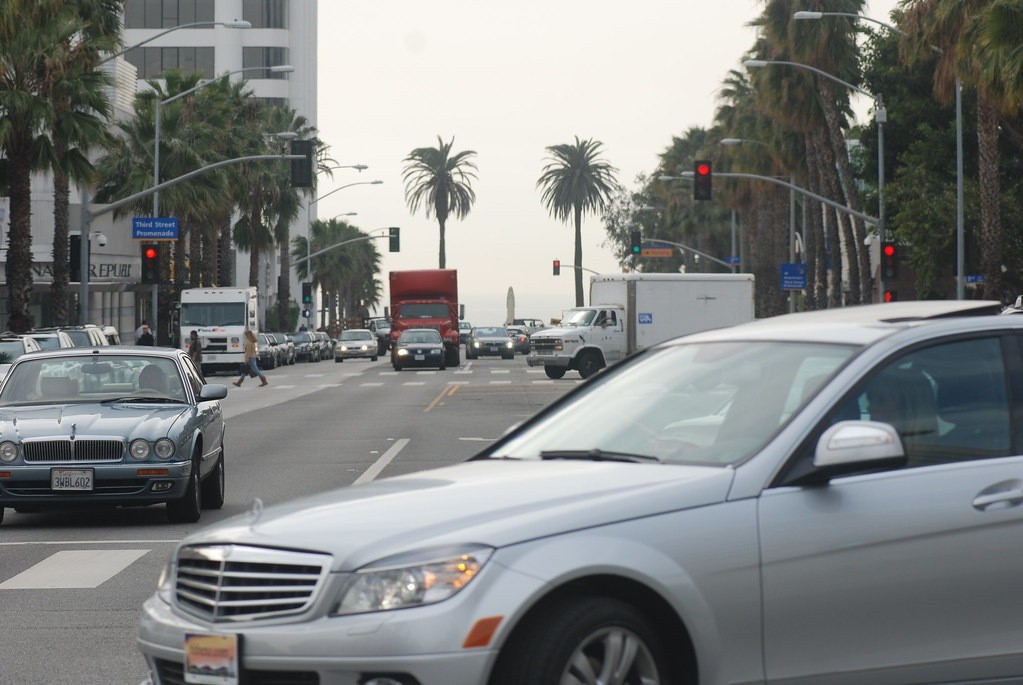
[
  {"x1": 299, "y1": 324, "x2": 307, "y2": 332},
  {"x1": 233, "y1": 330, "x2": 268, "y2": 387},
  {"x1": 866, "y1": 369, "x2": 939, "y2": 467},
  {"x1": 138, "y1": 365, "x2": 170, "y2": 397},
  {"x1": 135, "y1": 319, "x2": 154, "y2": 346},
  {"x1": 189, "y1": 330, "x2": 202, "y2": 371}
]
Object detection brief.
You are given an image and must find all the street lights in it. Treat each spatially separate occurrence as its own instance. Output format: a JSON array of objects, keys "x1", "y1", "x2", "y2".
[
  {"x1": 306, "y1": 165, "x2": 401, "y2": 329},
  {"x1": 794, "y1": 10, "x2": 964, "y2": 298},
  {"x1": 658, "y1": 60, "x2": 886, "y2": 300},
  {"x1": 76, "y1": 21, "x2": 312, "y2": 323}
]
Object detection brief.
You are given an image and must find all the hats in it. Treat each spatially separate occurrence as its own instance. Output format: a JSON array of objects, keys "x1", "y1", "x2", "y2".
[{"x1": 191, "y1": 330, "x2": 199, "y2": 337}]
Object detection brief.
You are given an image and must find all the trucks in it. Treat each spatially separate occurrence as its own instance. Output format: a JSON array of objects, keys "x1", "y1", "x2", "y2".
[
  {"x1": 363, "y1": 269, "x2": 464, "y2": 365},
  {"x1": 172, "y1": 287, "x2": 260, "y2": 375},
  {"x1": 527, "y1": 273, "x2": 755, "y2": 380}
]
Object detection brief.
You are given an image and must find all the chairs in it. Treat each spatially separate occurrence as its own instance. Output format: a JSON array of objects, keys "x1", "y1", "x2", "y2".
[
  {"x1": 48, "y1": 379, "x2": 82, "y2": 399},
  {"x1": 802, "y1": 375, "x2": 860, "y2": 421}
]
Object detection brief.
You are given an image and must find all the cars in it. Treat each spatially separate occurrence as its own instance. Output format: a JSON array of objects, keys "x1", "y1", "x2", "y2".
[
  {"x1": 392, "y1": 328, "x2": 446, "y2": 371},
  {"x1": 331, "y1": 329, "x2": 380, "y2": 363},
  {"x1": 0, "y1": 346, "x2": 227, "y2": 525},
  {"x1": 258, "y1": 331, "x2": 334, "y2": 369},
  {"x1": 0, "y1": 323, "x2": 121, "y2": 396},
  {"x1": 459, "y1": 319, "x2": 554, "y2": 360},
  {"x1": 136, "y1": 299, "x2": 1023, "y2": 685}
]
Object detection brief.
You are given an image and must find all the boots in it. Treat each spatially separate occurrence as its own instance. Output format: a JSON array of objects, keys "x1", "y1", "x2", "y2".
[
  {"x1": 232, "y1": 378, "x2": 244, "y2": 387},
  {"x1": 259, "y1": 377, "x2": 268, "y2": 386}
]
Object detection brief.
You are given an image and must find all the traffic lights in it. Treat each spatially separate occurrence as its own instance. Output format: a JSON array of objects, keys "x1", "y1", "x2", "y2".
[
  {"x1": 553, "y1": 261, "x2": 560, "y2": 275},
  {"x1": 694, "y1": 160, "x2": 712, "y2": 200},
  {"x1": 881, "y1": 242, "x2": 898, "y2": 301},
  {"x1": 631, "y1": 231, "x2": 642, "y2": 254},
  {"x1": 141, "y1": 245, "x2": 159, "y2": 284}
]
